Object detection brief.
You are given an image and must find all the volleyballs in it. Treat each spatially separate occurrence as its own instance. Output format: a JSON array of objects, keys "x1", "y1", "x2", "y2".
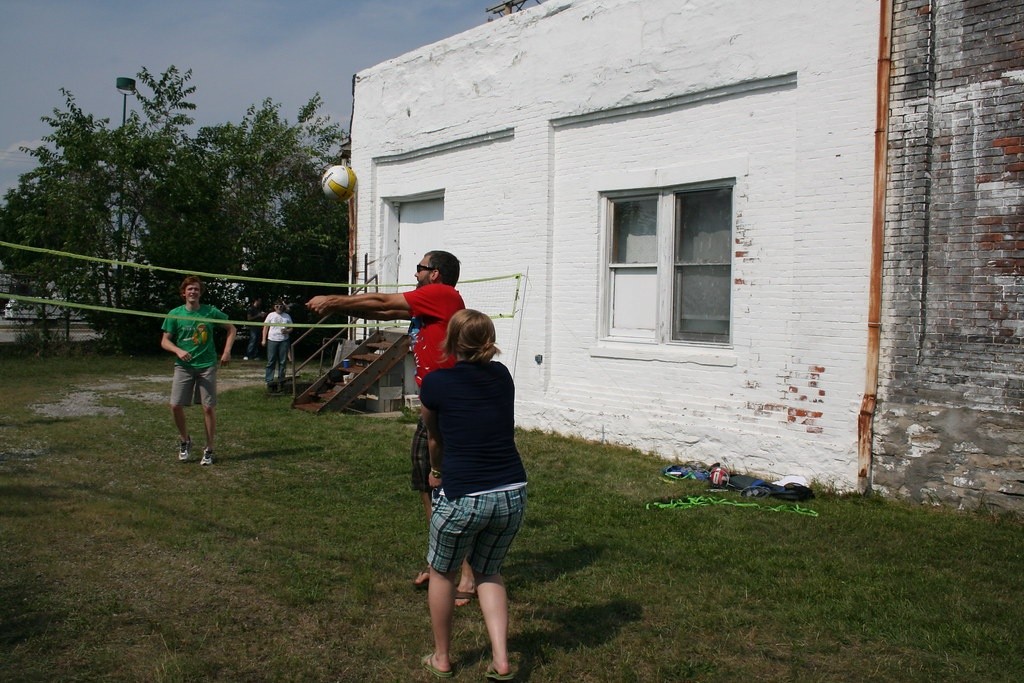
[
  {"x1": 710, "y1": 467, "x2": 731, "y2": 488},
  {"x1": 321, "y1": 164, "x2": 359, "y2": 201}
]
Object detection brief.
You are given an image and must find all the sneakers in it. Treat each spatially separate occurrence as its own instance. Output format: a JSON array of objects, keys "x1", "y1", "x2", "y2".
[
  {"x1": 200, "y1": 447, "x2": 212, "y2": 466},
  {"x1": 179, "y1": 435, "x2": 192, "y2": 461}
]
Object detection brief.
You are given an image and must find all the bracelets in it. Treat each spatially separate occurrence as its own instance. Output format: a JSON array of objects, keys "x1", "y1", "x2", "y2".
[{"x1": 431, "y1": 467, "x2": 440, "y2": 479}]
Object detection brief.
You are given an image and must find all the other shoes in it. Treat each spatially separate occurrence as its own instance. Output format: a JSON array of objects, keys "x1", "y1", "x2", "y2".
[
  {"x1": 267, "y1": 383, "x2": 275, "y2": 392},
  {"x1": 243, "y1": 357, "x2": 248, "y2": 360},
  {"x1": 255, "y1": 358, "x2": 260, "y2": 361},
  {"x1": 277, "y1": 382, "x2": 284, "y2": 393}
]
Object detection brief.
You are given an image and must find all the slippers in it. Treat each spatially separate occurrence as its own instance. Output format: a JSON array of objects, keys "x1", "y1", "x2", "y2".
[
  {"x1": 421, "y1": 654, "x2": 453, "y2": 678},
  {"x1": 454, "y1": 581, "x2": 477, "y2": 607},
  {"x1": 413, "y1": 563, "x2": 430, "y2": 590},
  {"x1": 485, "y1": 662, "x2": 514, "y2": 680}
]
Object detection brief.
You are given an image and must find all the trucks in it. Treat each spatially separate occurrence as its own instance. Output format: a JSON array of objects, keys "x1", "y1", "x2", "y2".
[{"x1": 3, "y1": 296, "x2": 82, "y2": 327}]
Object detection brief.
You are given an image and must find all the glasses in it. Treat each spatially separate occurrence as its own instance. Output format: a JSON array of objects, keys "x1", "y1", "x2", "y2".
[
  {"x1": 274, "y1": 304, "x2": 283, "y2": 309},
  {"x1": 417, "y1": 264, "x2": 441, "y2": 275}
]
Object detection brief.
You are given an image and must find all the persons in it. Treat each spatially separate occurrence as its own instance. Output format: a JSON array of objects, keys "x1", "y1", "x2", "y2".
[
  {"x1": 307, "y1": 251, "x2": 476, "y2": 607},
  {"x1": 419, "y1": 309, "x2": 529, "y2": 681},
  {"x1": 160, "y1": 276, "x2": 238, "y2": 466},
  {"x1": 261, "y1": 297, "x2": 293, "y2": 381},
  {"x1": 243, "y1": 297, "x2": 266, "y2": 361}
]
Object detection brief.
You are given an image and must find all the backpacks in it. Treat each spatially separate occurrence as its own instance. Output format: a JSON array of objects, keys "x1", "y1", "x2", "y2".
[{"x1": 663, "y1": 464, "x2": 709, "y2": 482}]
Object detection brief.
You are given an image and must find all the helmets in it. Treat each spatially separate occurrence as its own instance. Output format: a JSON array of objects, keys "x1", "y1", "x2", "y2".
[{"x1": 710, "y1": 466, "x2": 730, "y2": 488}]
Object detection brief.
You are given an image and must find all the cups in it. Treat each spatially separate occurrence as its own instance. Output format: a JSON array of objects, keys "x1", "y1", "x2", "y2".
[
  {"x1": 343, "y1": 359, "x2": 349, "y2": 368},
  {"x1": 281, "y1": 321, "x2": 286, "y2": 329}
]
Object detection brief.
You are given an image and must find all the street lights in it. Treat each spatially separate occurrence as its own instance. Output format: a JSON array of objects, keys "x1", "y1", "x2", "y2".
[{"x1": 114, "y1": 77, "x2": 136, "y2": 311}]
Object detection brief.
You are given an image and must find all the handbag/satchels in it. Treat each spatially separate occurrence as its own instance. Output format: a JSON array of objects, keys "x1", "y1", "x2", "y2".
[{"x1": 731, "y1": 474, "x2": 815, "y2": 501}]
